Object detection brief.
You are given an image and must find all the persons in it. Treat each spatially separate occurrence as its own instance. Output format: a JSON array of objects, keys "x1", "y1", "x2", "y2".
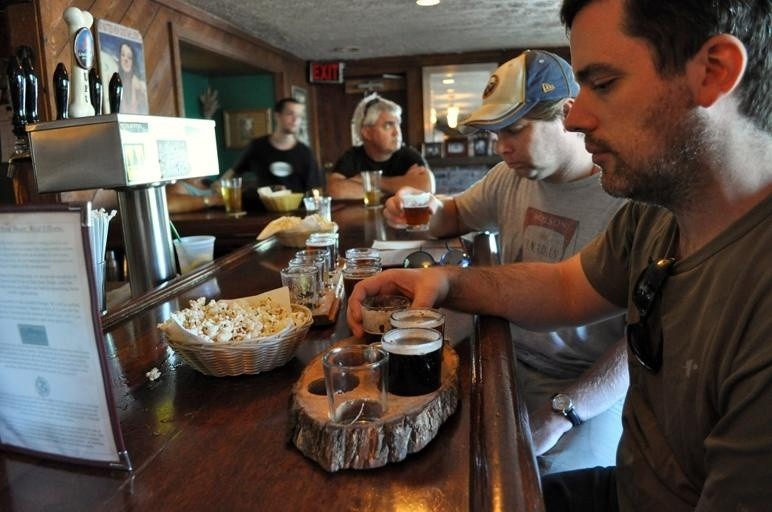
[
  {"x1": 168, "y1": 178, "x2": 225, "y2": 214},
  {"x1": 347, "y1": 0, "x2": 771, "y2": 511},
  {"x1": 217, "y1": 96, "x2": 323, "y2": 213},
  {"x1": 115, "y1": 42, "x2": 150, "y2": 121},
  {"x1": 382, "y1": 49, "x2": 631, "y2": 475},
  {"x1": 327, "y1": 94, "x2": 438, "y2": 201}
]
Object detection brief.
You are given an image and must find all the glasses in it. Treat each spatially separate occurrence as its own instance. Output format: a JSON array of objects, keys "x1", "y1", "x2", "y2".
[
  {"x1": 628, "y1": 257, "x2": 675, "y2": 373},
  {"x1": 404, "y1": 241, "x2": 471, "y2": 269}
]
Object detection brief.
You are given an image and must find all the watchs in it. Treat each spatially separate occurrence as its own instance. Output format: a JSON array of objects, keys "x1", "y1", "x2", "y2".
[{"x1": 551, "y1": 392, "x2": 584, "y2": 428}]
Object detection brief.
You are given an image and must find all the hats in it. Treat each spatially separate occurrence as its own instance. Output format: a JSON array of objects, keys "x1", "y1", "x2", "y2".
[{"x1": 461, "y1": 49, "x2": 581, "y2": 132}]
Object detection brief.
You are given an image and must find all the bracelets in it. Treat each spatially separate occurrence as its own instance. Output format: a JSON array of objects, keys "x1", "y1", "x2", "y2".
[{"x1": 201, "y1": 194, "x2": 210, "y2": 210}]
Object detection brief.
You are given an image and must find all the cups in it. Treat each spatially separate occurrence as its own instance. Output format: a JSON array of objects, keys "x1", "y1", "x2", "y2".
[
  {"x1": 94, "y1": 261, "x2": 108, "y2": 317},
  {"x1": 388, "y1": 306, "x2": 446, "y2": 344},
  {"x1": 179, "y1": 279, "x2": 221, "y2": 300},
  {"x1": 342, "y1": 247, "x2": 384, "y2": 298},
  {"x1": 304, "y1": 196, "x2": 332, "y2": 221},
  {"x1": 280, "y1": 232, "x2": 340, "y2": 310},
  {"x1": 365, "y1": 207, "x2": 387, "y2": 246},
  {"x1": 401, "y1": 189, "x2": 434, "y2": 233},
  {"x1": 381, "y1": 328, "x2": 444, "y2": 399},
  {"x1": 360, "y1": 169, "x2": 383, "y2": 209},
  {"x1": 322, "y1": 344, "x2": 390, "y2": 422},
  {"x1": 220, "y1": 176, "x2": 243, "y2": 213},
  {"x1": 104, "y1": 329, "x2": 131, "y2": 413},
  {"x1": 360, "y1": 295, "x2": 410, "y2": 343},
  {"x1": 174, "y1": 235, "x2": 215, "y2": 274}
]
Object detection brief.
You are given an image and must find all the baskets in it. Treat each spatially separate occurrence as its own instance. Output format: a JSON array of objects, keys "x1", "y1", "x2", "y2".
[
  {"x1": 275, "y1": 219, "x2": 337, "y2": 248},
  {"x1": 260, "y1": 191, "x2": 303, "y2": 211},
  {"x1": 163, "y1": 301, "x2": 313, "y2": 376}
]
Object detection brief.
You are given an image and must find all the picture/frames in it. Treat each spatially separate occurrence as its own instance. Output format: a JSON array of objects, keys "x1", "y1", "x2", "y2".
[{"x1": 223, "y1": 108, "x2": 273, "y2": 149}]
